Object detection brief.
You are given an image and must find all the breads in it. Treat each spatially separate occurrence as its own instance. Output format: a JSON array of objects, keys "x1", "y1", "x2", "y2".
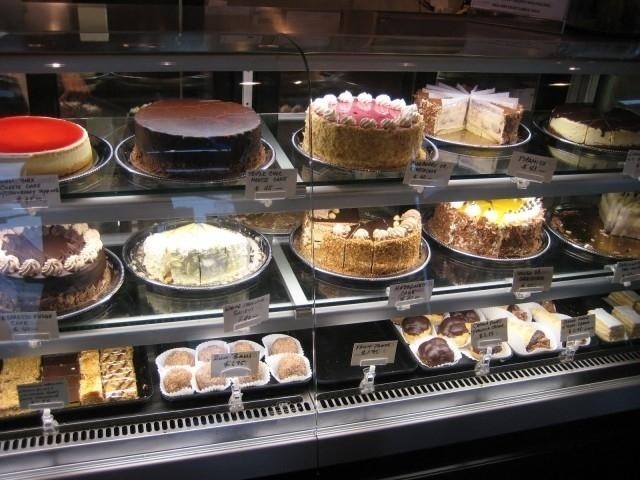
[
  {"x1": 402, "y1": 309, "x2": 503, "y2": 368},
  {"x1": 163, "y1": 336, "x2": 307, "y2": 395},
  {"x1": 507, "y1": 301, "x2": 587, "y2": 353},
  {"x1": 586, "y1": 289, "x2": 640, "y2": 344},
  {"x1": 1, "y1": 345, "x2": 140, "y2": 421}
]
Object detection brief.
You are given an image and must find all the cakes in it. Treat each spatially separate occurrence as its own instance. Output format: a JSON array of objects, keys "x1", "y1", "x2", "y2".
[
  {"x1": 599, "y1": 192, "x2": 640, "y2": 241},
  {"x1": 228, "y1": 212, "x2": 301, "y2": 231},
  {"x1": 137, "y1": 284, "x2": 251, "y2": 314},
  {"x1": 299, "y1": 205, "x2": 422, "y2": 277},
  {"x1": 430, "y1": 196, "x2": 548, "y2": 258},
  {"x1": 545, "y1": 101, "x2": 640, "y2": 151},
  {"x1": 0, "y1": 223, "x2": 113, "y2": 316},
  {"x1": 130, "y1": 99, "x2": 266, "y2": 179},
  {"x1": 414, "y1": 82, "x2": 525, "y2": 145},
  {"x1": 141, "y1": 222, "x2": 261, "y2": 287},
  {"x1": 302, "y1": 90, "x2": 426, "y2": 168},
  {"x1": 0, "y1": 114, "x2": 94, "y2": 181}
]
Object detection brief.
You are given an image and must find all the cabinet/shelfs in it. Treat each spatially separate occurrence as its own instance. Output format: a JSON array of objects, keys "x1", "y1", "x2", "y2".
[{"x1": 0, "y1": 29, "x2": 640, "y2": 480}]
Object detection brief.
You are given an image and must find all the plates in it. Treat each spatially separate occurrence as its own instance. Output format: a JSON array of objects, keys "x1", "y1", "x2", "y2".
[
  {"x1": 289, "y1": 250, "x2": 430, "y2": 294},
  {"x1": 425, "y1": 204, "x2": 639, "y2": 264},
  {"x1": 124, "y1": 214, "x2": 274, "y2": 292},
  {"x1": 0, "y1": 245, "x2": 124, "y2": 321},
  {"x1": 116, "y1": 135, "x2": 275, "y2": 182},
  {"x1": 291, "y1": 110, "x2": 533, "y2": 170},
  {"x1": 291, "y1": 218, "x2": 432, "y2": 281},
  {"x1": 558, "y1": 242, "x2": 612, "y2": 265},
  {"x1": 434, "y1": 247, "x2": 543, "y2": 271},
  {"x1": 532, "y1": 103, "x2": 638, "y2": 158},
  {"x1": 56, "y1": 131, "x2": 113, "y2": 184}
]
